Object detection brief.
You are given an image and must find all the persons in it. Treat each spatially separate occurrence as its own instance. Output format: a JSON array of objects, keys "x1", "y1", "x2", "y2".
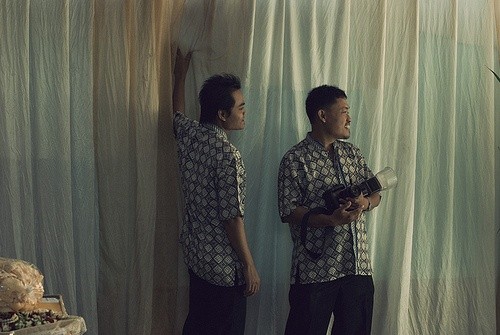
[
  {"x1": 171, "y1": 44, "x2": 261, "y2": 335},
  {"x1": 277, "y1": 85, "x2": 382, "y2": 335}
]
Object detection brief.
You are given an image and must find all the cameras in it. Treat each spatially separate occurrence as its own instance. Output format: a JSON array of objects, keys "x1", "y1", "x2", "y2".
[{"x1": 322, "y1": 166, "x2": 399, "y2": 216}]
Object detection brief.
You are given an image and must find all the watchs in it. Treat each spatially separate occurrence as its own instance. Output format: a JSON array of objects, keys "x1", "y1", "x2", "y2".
[{"x1": 366, "y1": 197, "x2": 371, "y2": 212}]
